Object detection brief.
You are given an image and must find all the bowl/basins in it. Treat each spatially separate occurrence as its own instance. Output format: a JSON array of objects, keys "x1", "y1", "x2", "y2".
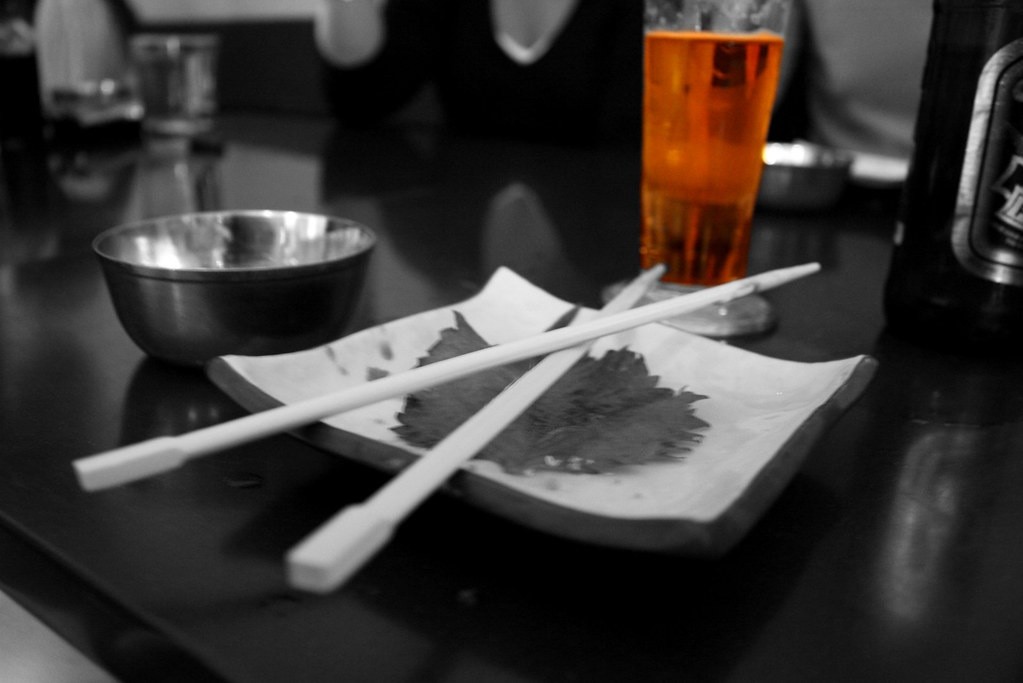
[
  {"x1": 758, "y1": 137, "x2": 854, "y2": 220},
  {"x1": 89, "y1": 206, "x2": 378, "y2": 365}
]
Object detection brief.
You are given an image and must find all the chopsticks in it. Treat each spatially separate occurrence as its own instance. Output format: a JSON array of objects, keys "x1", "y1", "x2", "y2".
[{"x1": 75, "y1": 263, "x2": 822, "y2": 591}]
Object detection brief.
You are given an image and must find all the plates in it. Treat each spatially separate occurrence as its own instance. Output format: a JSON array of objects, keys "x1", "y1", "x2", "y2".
[{"x1": 208, "y1": 263, "x2": 878, "y2": 548}]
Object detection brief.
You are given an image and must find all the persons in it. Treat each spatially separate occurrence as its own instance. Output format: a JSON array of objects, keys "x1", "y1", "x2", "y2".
[
  {"x1": 314, "y1": 0, "x2": 643, "y2": 122},
  {"x1": 777, "y1": 0, "x2": 932, "y2": 179}
]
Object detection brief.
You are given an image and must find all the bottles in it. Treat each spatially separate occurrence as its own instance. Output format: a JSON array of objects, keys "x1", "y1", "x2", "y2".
[{"x1": 881, "y1": 0, "x2": 1023, "y2": 366}]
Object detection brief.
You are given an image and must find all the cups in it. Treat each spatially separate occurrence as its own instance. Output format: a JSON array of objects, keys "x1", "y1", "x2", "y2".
[
  {"x1": 127, "y1": 31, "x2": 219, "y2": 137},
  {"x1": 637, "y1": 0, "x2": 786, "y2": 285}
]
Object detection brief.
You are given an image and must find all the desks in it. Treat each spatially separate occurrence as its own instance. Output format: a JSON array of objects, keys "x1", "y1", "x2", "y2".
[{"x1": 0, "y1": 117, "x2": 1023, "y2": 682}]
